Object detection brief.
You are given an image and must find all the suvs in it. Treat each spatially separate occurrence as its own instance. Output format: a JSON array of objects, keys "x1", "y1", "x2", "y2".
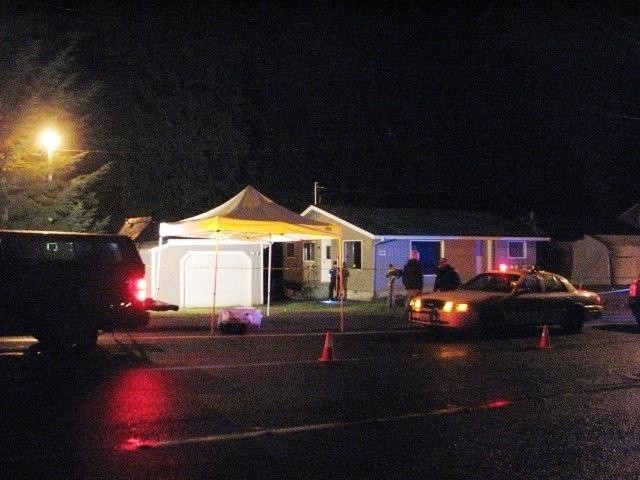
[{"x1": 1, "y1": 230, "x2": 153, "y2": 353}]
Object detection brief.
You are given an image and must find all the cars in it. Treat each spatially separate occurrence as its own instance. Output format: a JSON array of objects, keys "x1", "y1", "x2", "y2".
[
  {"x1": 629, "y1": 276, "x2": 640, "y2": 327},
  {"x1": 404, "y1": 264, "x2": 605, "y2": 337}
]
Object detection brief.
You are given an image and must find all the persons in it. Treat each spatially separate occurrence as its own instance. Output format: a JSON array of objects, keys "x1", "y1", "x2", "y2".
[
  {"x1": 328, "y1": 260, "x2": 340, "y2": 302},
  {"x1": 401, "y1": 250, "x2": 422, "y2": 312},
  {"x1": 336, "y1": 261, "x2": 350, "y2": 301},
  {"x1": 432, "y1": 257, "x2": 462, "y2": 292}
]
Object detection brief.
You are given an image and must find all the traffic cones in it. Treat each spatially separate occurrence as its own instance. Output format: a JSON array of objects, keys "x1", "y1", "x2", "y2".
[
  {"x1": 534, "y1": 321, "x2": 555, "y2": 353},
  {"x1": 313, "y1": 332, "x2": 342, "y2": 365}
]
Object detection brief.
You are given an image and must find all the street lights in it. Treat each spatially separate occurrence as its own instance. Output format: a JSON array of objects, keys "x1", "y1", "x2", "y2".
[{"x1": 37, "y1": 126, "x2": 62, "y2": 230}]
